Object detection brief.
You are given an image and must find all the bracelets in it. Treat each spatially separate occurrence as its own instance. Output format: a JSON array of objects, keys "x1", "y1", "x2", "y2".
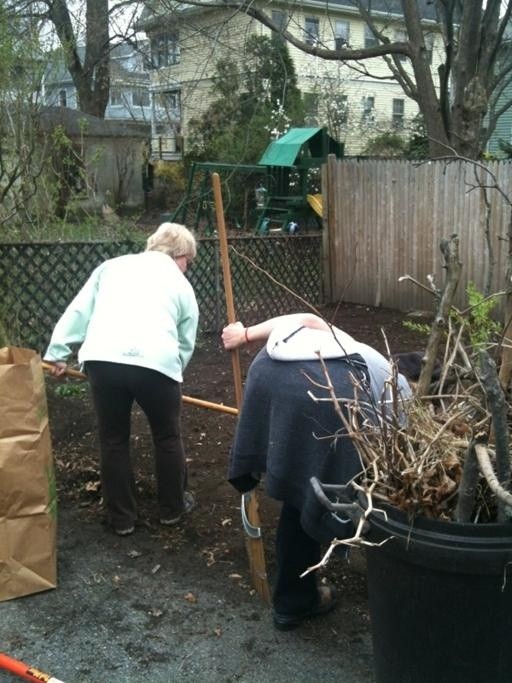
[{"x1": 245, "y1": 326, "x2": 251, "y2": 344}]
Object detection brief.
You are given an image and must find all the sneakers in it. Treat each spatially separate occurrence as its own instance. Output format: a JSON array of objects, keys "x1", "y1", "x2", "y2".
[
  {"x1": 115, "y1": 523, "x2": 135, "y2": 536},
  {"x1": 160, "y1": 491, "x2": 195, "y2": 525},
  {"x1": 272, "y1": 584, "x2": 338, "y2": 631}
]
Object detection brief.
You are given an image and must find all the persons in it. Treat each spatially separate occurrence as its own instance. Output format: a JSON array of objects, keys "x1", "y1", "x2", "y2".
[
  {"x1": 222, "y1": 313, "x2": 446, "y2": 631},
  {"x1": 43, "y1": 221, "x2": 199, "y2": 533}
]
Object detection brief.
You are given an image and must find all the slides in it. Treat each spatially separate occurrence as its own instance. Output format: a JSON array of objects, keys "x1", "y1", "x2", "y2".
[{"x1": 307, "y1": 192, "x2": 321, "y2": 218}]
